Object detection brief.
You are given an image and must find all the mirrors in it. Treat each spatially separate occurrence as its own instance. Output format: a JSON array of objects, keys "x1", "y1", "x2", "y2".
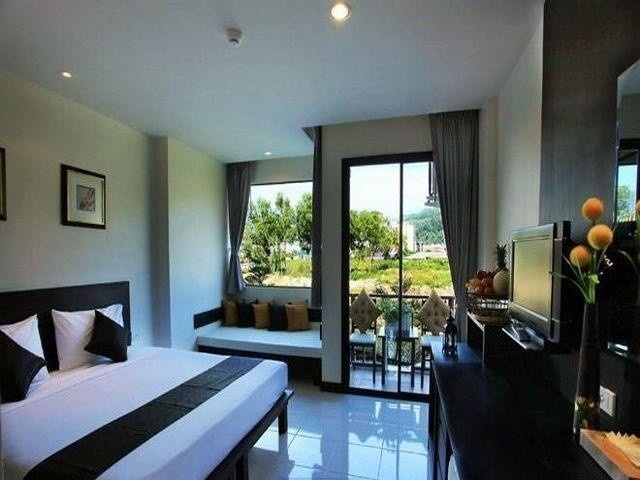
[{"x1": 610, "y1": 56, "x2": 640, "y2": 245}]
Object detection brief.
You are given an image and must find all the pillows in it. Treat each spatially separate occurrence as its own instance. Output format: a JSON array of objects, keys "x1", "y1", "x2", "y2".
[
  {"x1": 350, "y1": 287, "x2": 383, "y2": 332},
  {"x1": 221, "y1": 299, "x2": 311, "y2": 332},
  {"x1": 0, "y1": 303, "x2": 129, "y2": 406},
  {"x1": 417, "y1": 289, "x2": 454, "y2": 336}
]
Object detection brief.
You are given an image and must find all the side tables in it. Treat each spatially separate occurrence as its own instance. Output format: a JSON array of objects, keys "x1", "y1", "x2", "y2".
[{"x1": 376, "y1": 327, "x2": 419, "y2": 388}]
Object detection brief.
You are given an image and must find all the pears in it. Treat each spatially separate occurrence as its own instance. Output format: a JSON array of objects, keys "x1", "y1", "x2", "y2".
[
  {"x1": 485, "y1": 287, "x2": 493, "y2": 295},
  {"x1": 475, "y1": 287, "x2": 483, "y2": 292}
]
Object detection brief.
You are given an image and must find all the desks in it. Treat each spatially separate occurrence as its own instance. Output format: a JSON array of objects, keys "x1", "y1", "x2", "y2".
[{"x1": 426, "y1": 341, "x2": 640, "y2": 480}]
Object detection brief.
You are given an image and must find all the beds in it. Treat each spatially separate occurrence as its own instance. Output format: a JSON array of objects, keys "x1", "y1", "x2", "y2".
[{"x1": 0, "y1": 279, "x2": 295, "y2": 478}]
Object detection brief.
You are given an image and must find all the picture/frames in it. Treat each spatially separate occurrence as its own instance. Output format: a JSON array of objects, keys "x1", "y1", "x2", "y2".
[{"x1": 0, "y1": 146, "x2": 106, "y2": 228}]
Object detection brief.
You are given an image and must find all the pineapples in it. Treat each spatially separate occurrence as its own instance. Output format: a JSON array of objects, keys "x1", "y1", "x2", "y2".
[
  {"x1": 482, "y1": 277, "x2": 492, "y2": 288},
  {"x1": 495, "y1": 244, "x2": 508, "y2": 273}
]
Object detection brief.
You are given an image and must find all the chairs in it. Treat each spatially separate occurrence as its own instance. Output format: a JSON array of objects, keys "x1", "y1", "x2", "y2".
[
  {"x1": 422, "y1": 294, "x2": 455, "y2": 389},
  {"x1": 350, "y1": 297, "x2": 377, "y2": 370}
]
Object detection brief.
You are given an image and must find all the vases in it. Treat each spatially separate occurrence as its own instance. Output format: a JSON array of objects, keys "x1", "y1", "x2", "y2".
[{"x1": 573, "y1": 302, "x2": 603, "y2": 435}]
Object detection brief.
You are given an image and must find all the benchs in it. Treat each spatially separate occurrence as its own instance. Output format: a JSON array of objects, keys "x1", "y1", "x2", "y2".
[{"x1": 197, "y1": 305, "x2": 322, "y2": 383}]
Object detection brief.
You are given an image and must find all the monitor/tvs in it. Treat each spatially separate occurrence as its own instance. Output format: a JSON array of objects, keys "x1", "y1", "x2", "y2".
[{"x1": 507, "y1": 219, "x2": 572, "y2": 344}]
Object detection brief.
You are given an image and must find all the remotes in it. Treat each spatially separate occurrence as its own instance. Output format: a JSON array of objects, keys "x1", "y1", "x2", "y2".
[{"x1": 511, "y1": 322, "x2": 531, "y2": 341}]
